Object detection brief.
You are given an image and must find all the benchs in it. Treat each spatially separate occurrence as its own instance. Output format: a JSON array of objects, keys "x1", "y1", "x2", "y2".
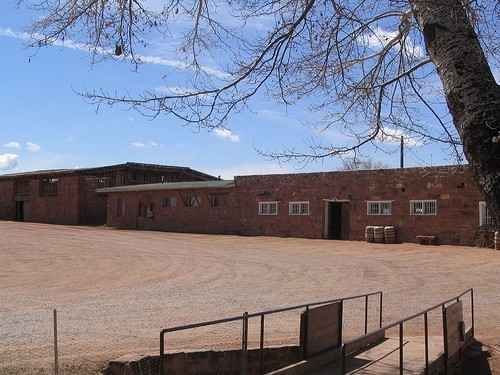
[{"x1": 416, "y1": 233, "x2": 440, "y2": 245}]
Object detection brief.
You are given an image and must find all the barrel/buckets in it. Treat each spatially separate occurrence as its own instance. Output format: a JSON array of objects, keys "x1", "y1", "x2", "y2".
[
  {"x1": 384, "y1": 226, "x2": 396, "y2": 244},
  {"x1": 364, "y1": 225, "x2": 374, "y2": 242},
  {"x1": 373, "y1": 226, "x2": 385, "y2": 242}
]
